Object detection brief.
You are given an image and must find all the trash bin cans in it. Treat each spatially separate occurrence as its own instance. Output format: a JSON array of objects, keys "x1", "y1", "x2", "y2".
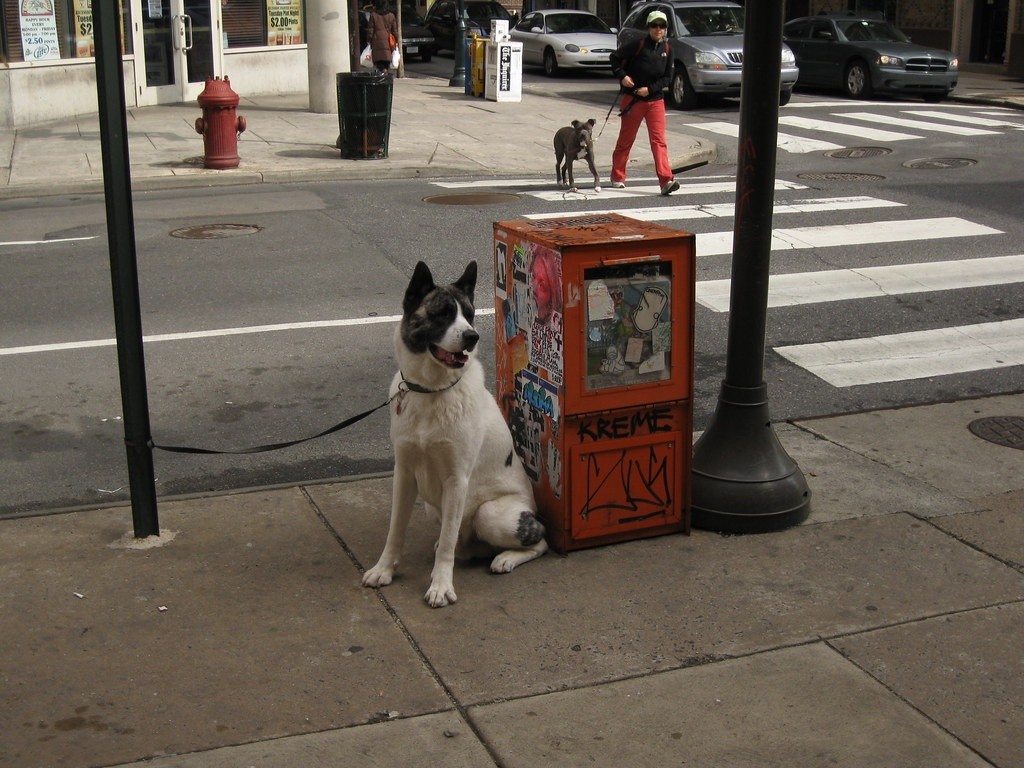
[
  {"x1": 461, "y1": 19, "x2": 525, "y2": 102},
  {"x1": 492, "y1": 209, "x2": 699, "y2": 553},
  {"x1": 333, "y1": 68, "x2": 395, "y2": 162}
]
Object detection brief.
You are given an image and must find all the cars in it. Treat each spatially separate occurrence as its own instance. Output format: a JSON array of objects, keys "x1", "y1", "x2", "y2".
[
  {"x1": 423, "y1": 0, "x2": 520, "y2": 54},
  {"x1": 357, "y1": 0, "x2": 436, "y2": 64},
  {"x1": 508, "y1": 8, "x2": 620, "y2": 78},
  {"x1": 778, "y1": 14, "x2": 960, "y2": 104}
]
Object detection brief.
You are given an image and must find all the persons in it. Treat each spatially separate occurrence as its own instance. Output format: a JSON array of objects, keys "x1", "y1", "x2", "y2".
[
  {"x1": 609, "y1": 11, "x2": 680, "y2": 196},
  {"x1": 366, "y1": 0, "x2": 398, "y2": 75}
]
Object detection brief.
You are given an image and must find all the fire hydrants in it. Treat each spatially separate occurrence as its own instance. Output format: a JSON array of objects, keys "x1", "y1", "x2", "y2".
[{"x1": 194, "y1": 74, "x2": 248, "y2": 171}]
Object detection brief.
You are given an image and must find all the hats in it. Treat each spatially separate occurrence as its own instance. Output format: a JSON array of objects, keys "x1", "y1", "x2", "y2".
[{"x1": 646, "y1": 10, "x2": 668, "y2": 27}]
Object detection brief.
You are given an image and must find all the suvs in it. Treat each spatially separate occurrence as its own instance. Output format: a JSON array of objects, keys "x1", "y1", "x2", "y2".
[{"x1": 611, "y1": 0, "x2": 800, "y2": 110}]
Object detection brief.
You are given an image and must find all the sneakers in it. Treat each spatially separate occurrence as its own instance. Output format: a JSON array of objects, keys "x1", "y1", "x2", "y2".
[
  {"x1": 610, "y1": 176, "x2": 626, "y2": 188},
  {"x1": 661, "y1": 180, "x2": 680, "y2": 195}
]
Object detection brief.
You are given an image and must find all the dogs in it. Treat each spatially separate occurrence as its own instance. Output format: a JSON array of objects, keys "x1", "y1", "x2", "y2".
[
  {"x1": 361, "y1": 259, "x2": 549, "y2": 610},
  {"x1": 553, "y1": 117, "x2": 602, "y2": 193}
]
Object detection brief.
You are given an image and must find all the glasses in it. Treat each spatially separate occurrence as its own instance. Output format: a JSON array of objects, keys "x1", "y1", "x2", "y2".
[{"x1": 648, "y1": 22, "x2": 668, "y2": 29}]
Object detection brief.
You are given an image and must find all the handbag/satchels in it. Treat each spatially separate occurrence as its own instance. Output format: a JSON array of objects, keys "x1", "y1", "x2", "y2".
[
  {"x1": 388, "y1": 31, "x2": 396, "y2": 50},
  {"x1": 390, "y1": 42, "x2": 400, "y2": 71},
  {"x1": 359, "y1": 42, "x2": 375, "y2": 69}
]
